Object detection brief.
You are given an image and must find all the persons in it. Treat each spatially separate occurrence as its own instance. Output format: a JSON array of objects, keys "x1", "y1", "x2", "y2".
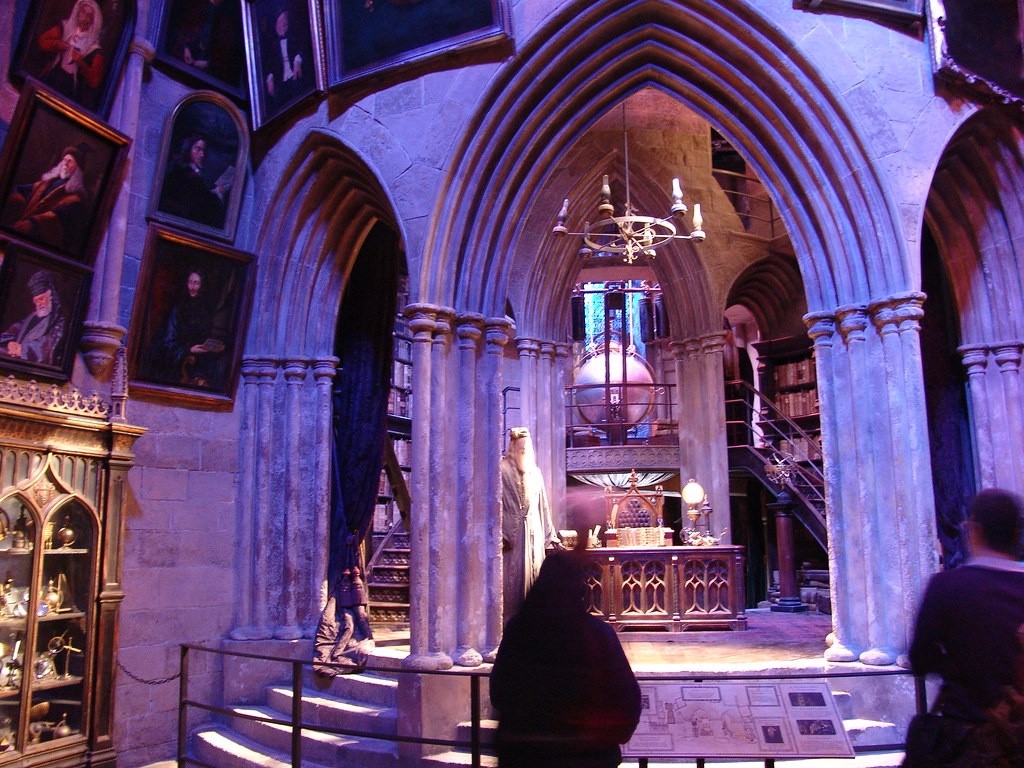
[
  {"x1": 502, "y1": 426, "x2": 566, "y2": 628},
  {"x1": 489, "y1": 551, "x2": 641, "y2": 768},
  {"x1": 905, "y1": 487, "x2": 1024, "y2": 768}
]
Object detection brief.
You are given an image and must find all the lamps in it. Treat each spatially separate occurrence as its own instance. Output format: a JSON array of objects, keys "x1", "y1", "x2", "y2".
[{"x1": 554, "y1": 99, "x2": 707, "y2": 263}]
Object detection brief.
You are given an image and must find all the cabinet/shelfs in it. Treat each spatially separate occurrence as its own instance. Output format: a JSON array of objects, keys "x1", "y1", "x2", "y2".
[{"x1": 0, "y1": 371, "x2": 146, "y2": 768}]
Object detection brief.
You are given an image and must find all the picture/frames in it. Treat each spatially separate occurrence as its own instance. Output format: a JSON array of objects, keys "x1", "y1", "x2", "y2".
[
  {"x1": 1, "y1": 77, "x2": 133, "y2": 270},
  {"x1": 150, "y1": 0, "x2": 250, "y2": 105},
  {"x1": 326, "y1": 0, "x2": 516, "y2": 92},
  {"x1": 0, "y1": 235, "x2": 95, "y2": 383},
  {"x1": 6, "y1": 0, "x2": 140, "y2": 120},
  {"x1": 238, "y1": 0, "x2": 327, "y2": 136},
  {"x1": 120, "y1": 222, "x2": 259, "y2": 412},
  {"x1": 143, "y1": 87, "x2": 252, "y2": 245}
]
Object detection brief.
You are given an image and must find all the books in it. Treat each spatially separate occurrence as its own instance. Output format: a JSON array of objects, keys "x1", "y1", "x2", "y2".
[
  {"x1": 767, "y1": 355, "x2": 823, "y2": 462},
  {"x1": 373, "y1": 277, "x2": 412, "y2": 534}
]
